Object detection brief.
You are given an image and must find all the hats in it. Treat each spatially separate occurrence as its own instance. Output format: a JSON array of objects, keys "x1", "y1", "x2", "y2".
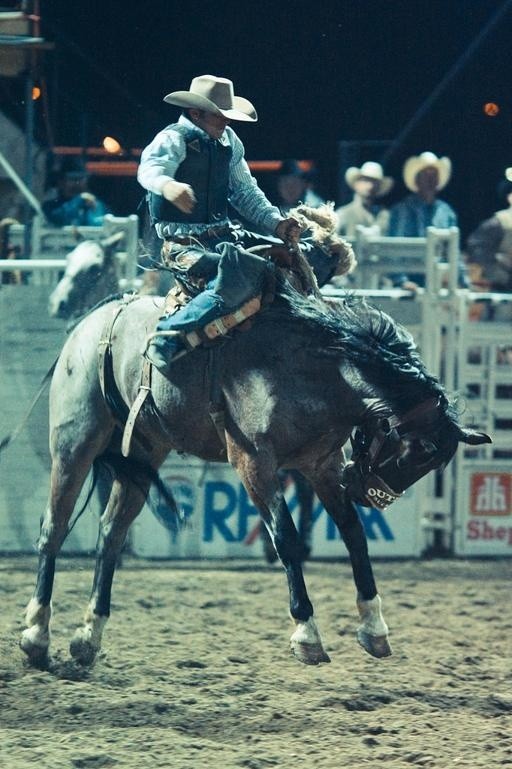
[
  {"x1": 402, "y1": 149, "x2": 451, "y2": 192},
  {"x1": 163, "y1": 75, "x2": 258, "y2": 124},
  {"x1": 345, "y1": 161, "x2": 392, "y2": 198}
]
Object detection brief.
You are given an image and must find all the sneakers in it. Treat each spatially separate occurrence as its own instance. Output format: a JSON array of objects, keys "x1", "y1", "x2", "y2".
[{"x1": 148, "y1": 345, "x2": 178, "y2": 375}]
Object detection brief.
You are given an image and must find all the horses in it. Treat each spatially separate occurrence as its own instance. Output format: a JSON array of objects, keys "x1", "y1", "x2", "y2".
[{"x1": 17, "y1": 287, "x2": 494, "y2": 673}]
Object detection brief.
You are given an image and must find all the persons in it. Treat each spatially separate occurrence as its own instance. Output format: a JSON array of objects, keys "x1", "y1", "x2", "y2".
[
  {"x1": 41, "y1": 155, "x2": 107, "y2": 227},
  {"x1": 137, "y1": 71, "x2": 307, "y2": 377},
  {"x1": 329, "y1": 152, "x2": 468, "y2": 300},
  {"x1": 269, "y1": 154, "x2": 322, "y2": 217},
  {"x1": 467, "y1": 166, "x2": 512, "y2": 320}
]
